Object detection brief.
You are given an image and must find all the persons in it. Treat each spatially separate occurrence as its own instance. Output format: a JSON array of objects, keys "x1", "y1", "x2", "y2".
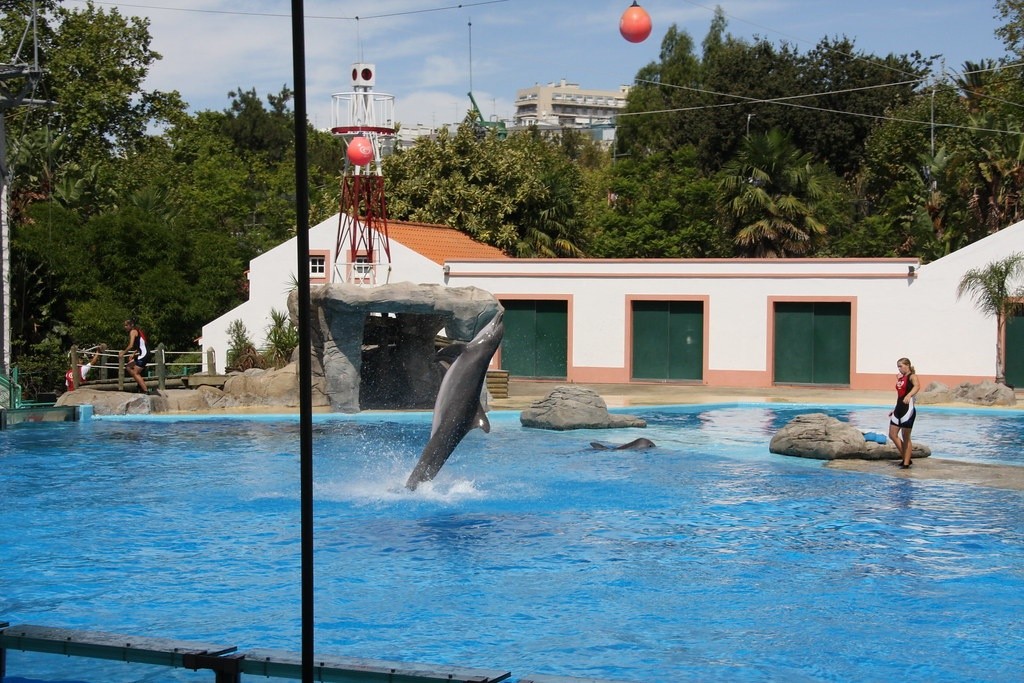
[
  {"x1": 889, "y1": 358, "x2": 921, "y2": 469},
  {"x1": 65, "y1": 349, "x2": 99, "y2": 392},
  {"x1": 119, "y1": 319, "x2": 150, "y2": 396}
]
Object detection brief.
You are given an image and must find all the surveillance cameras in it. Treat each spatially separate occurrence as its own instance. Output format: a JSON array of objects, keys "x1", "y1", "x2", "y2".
[
  {"x1": 908, "y1": 264, "x2": 920, "y2": 273},
  {"x1": 443, "y1": 265, "x2": 450, "y2": 273}
]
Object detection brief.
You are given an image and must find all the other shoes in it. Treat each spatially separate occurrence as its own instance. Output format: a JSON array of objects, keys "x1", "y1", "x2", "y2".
[
  {"x1": 142, "y1": 391, "x2": 150, "y2": 395},
  {"x1": 898, "y1": 459, "x2": 913, "y2": 470}
]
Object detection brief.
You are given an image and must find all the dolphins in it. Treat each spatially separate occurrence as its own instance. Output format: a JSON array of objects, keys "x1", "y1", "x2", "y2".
[
  {"x1": 590, "y1": 437, "x2": 655, "y2": 451},
  {"x1": 405, "y1": 308, "x2": 505, "y2": 490}
]
